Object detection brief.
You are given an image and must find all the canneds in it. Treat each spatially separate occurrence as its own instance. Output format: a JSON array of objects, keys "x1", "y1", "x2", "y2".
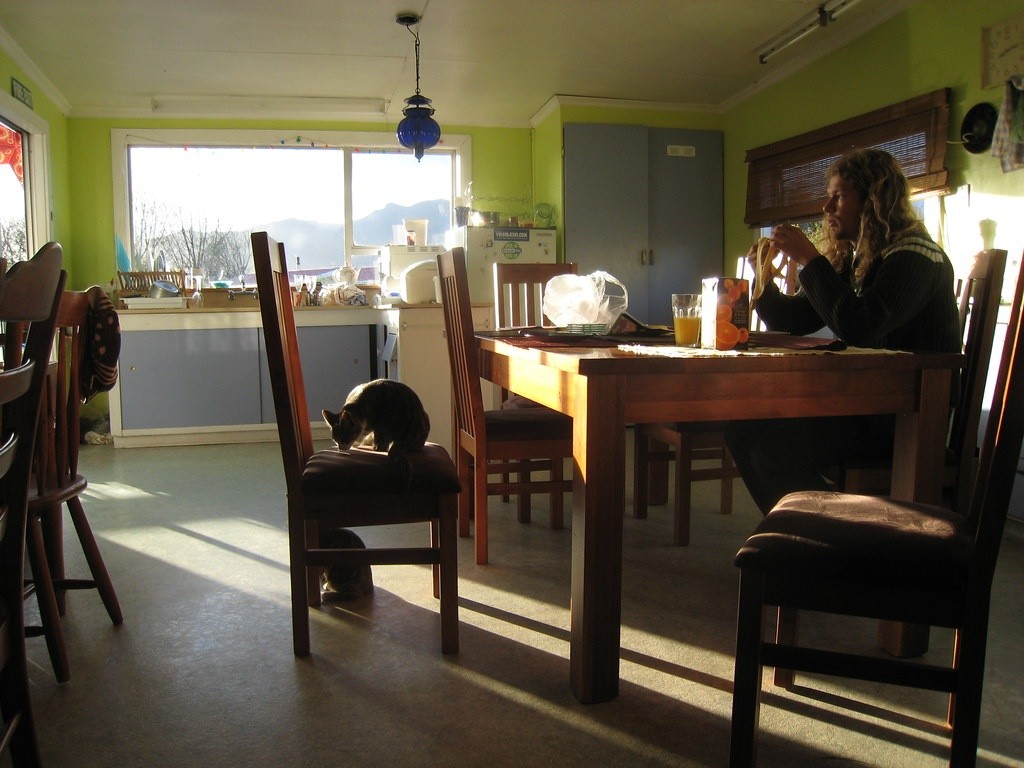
[{"x1": 508, "y1": 217, "x2": 518, "y2": 227}]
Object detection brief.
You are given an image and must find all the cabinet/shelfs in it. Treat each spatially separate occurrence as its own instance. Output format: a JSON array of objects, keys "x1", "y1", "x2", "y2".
[
  {"x1": 381, "y1": 307, "x2": 490, "y2": 458},
  {"x1": 530, "y1": 95, "x2": 726, "y2": 325},
  {"x1": 110, "y1": 314, "x2": 388, "y2": 448}
]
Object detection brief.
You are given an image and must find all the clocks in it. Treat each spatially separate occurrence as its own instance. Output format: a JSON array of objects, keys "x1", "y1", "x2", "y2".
[{"x1": 981, "y1": 10, "x2": 1024, "y2": 90}]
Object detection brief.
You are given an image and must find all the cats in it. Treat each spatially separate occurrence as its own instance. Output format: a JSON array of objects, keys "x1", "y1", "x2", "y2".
[
  {"x1": 321, "y1": 378, "x2": 431, "y2": 498},
  {"x1": 320, "y1": 528, "x2": 374, "y2": 602}
]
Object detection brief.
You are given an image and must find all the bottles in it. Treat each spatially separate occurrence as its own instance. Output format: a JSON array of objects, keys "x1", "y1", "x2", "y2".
[
  {"x1": 509, "y1": 204, "x2": 553, "y2": 228},
  {"x1": 190, "y1": 276, "x2": 205, "y2": 308},
  {"x1": 406, "y1": 230, "x2": 416, "y2": 246},
  {"x1": 105, "y1": 279, "x2": 124, "y2": 309},
  {"x1": 290, "y1": 282, "x2": 322, "y2": 307}
]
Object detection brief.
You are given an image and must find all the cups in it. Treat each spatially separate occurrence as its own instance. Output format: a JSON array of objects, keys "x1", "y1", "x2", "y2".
[
  {"x1": 294, "y1": 274, "x2": 303, "y2": 291},
  {"x1": 305, "y1": 276, "x2": 316, "y2": 293},
  {"x1": 672, "y1": 294, "x2": 702, "y2": 347},
  {"x1": 433, "y1": 277, "x2": 442, "y2": 303},
  {"x1": 455, "y1": 206, "x2": 499, "y2": 226},
  {"x1": 373, "y1": 294, "x2": 380, "y2": 308}
]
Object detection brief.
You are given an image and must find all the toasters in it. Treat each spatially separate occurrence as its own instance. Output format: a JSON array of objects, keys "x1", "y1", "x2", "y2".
[{"x1": 400, "y1": 260, "x2": 438, "y2": 304}]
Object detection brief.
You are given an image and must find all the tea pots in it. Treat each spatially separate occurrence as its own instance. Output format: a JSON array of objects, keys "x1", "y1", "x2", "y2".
[{"x1": 332, "y1": 263, "x2": 361, "y2": 285}]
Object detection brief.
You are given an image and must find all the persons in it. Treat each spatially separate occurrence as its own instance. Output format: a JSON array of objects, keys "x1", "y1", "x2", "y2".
[{"x1": 721, "y1": 148, "x2": 961, "y2": 517}]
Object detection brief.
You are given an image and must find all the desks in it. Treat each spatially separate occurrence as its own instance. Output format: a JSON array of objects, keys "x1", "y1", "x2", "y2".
[{"x1": 443, "y1": 325, "x2": 967, "y2": 704}]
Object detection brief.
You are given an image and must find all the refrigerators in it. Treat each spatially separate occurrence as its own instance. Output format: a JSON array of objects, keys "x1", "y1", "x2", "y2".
[{"x1": 445, "y1": 226, "x2": 556, "y2": 340}]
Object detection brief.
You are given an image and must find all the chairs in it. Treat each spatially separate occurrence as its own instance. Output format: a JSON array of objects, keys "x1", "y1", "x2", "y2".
[
  {"x1": 728, "y1": 248, "x2": 1024, "y2": 768},
  {"x1": 0, "y1": 242, "x2": 123, "y2": 768},
  {"x1": 436, "y1": 246, "x2": 578, "y2": 565},
  {"x1": 250, "y1": 231, "x2": 463, "y2": 658}
]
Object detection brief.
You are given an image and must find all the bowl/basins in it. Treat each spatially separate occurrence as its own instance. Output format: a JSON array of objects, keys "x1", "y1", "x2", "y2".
[{"x1": 149, "y1": 280, "x2": 178, "y2": 297}]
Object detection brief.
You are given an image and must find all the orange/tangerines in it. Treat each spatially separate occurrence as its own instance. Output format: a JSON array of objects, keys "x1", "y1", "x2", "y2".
[{"x1": 716, "y1": 279, "x2": 749, "y2": 350}]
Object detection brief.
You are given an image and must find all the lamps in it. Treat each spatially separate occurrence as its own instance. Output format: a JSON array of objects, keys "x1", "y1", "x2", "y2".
[
  {"x1": 753, "y1": 0, "x2": 861, "y2": 65},
  {"x1": 396, "y1": 13, "x2": 440, "y2": 163}
]
